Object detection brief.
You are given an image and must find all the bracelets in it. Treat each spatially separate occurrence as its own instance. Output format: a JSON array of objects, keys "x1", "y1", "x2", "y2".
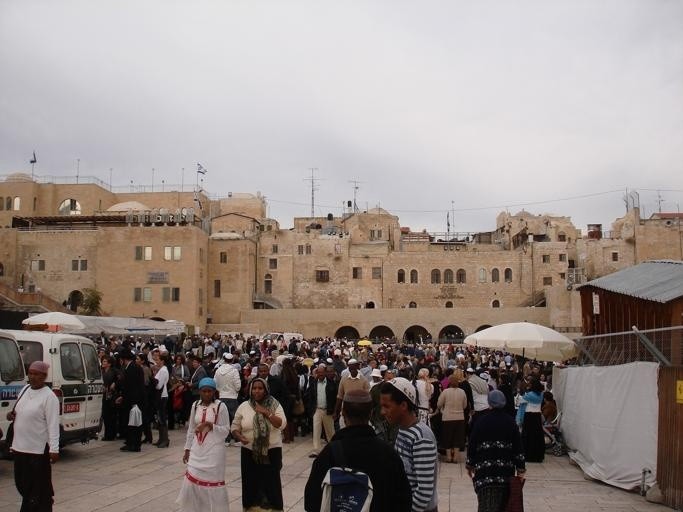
[{"x1": 267, "y1": 412, "x2": 273, "y2": 419}]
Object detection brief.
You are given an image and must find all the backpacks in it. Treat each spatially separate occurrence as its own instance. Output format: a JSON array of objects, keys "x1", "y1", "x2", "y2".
[{"x1": 319, "y1": 464, "x2": 374, "y2": 512}]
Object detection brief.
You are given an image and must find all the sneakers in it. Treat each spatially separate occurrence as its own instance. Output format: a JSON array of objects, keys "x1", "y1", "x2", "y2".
[
  {"x1": 152, "y1": 439, "x2": 171, "y2": 448},
  {"x1": 309, "y1": 452, "x2": 319, "y2": 459},
  {"x1": 119, "y1": 441, "x2": 141, "y2": 453},
  {"x1": 507, "y1": 474, "x2": 527, "y2": 512}
]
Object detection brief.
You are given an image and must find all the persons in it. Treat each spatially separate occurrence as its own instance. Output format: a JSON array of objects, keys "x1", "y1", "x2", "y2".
[
  {"x1": 6, "y1": 358, "x2": 62, "y2": 512},
  {"x1": 83, "y1": 331, "x2": 562, "y2": 462},
  {"x1": 463, "y1": 387, "x2": 526, "y2": 512},
  {"x1": 228, "y1": 376, "x2": 288, "y2": 512},
  {"x1": 374, "y1": 376, "x2": 441, "y2": 512},
  {"x1": 302, "y1": 388, "x2": 414, "y2": 512},
  {"x1": 174, "y1": 375, "x2": 231, "y2": 512}
]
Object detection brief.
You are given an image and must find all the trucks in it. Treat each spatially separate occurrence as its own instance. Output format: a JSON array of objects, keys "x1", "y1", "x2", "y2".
[{"x1": 260, "y1": 331, "x2": 304, "y2": 346}]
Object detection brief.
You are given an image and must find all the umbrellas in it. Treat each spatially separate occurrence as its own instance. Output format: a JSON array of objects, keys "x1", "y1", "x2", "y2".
[
  {"x1": 460, "y1": 318, "x2": 581, "y2": 393},
  {"x1": 21, "y1": 309, "x2": 83, "y2": 334}
]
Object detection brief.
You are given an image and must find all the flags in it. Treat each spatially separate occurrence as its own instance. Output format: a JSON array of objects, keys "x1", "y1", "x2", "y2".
[
  {"x1": 196, "y1": 161, "x2": 208, "y2": 174},
  {"x1": 28, "y1": 148, "x2": 38, "y2": 163},
  {"x1": 192, "y1": 192, "x2": 203, "y2": 211}
]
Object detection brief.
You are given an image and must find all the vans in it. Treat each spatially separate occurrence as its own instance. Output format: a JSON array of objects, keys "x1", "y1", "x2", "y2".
[
  {"x1": 0, "y1": 330, "x2": 27, "y2": 444},
  {"x1": 2, "y1": 328, "x2": 106, "y2": 448}
]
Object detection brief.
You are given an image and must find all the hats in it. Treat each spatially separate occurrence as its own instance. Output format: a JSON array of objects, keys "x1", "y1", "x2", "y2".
[
  {"x1": 198, "y1": 377, "x2": 217, "y2": 390},
  {"x1": 387, "y1": 376, "x2": 417, "y2": 405},
  {"x1": 464, "y1": 366, "x2": 491, "y2": 382},
  {"x1": 29, "y1": 361, "x2": 50, "y2": 375},
  {"x1": 110, "y1": 347, "x2": 256, "y2": 363},
  {"x1": 315, "y1": 356, "x2": 387, "y2": 379}
]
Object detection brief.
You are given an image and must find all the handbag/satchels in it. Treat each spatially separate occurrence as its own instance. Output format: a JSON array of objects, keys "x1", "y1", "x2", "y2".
[
  {"x1": 5, "y1": 422, "x2": 16, "y2": 446},
  {"x1": 129, "y1": 404, "x2": 142, "y2": 426}
]
